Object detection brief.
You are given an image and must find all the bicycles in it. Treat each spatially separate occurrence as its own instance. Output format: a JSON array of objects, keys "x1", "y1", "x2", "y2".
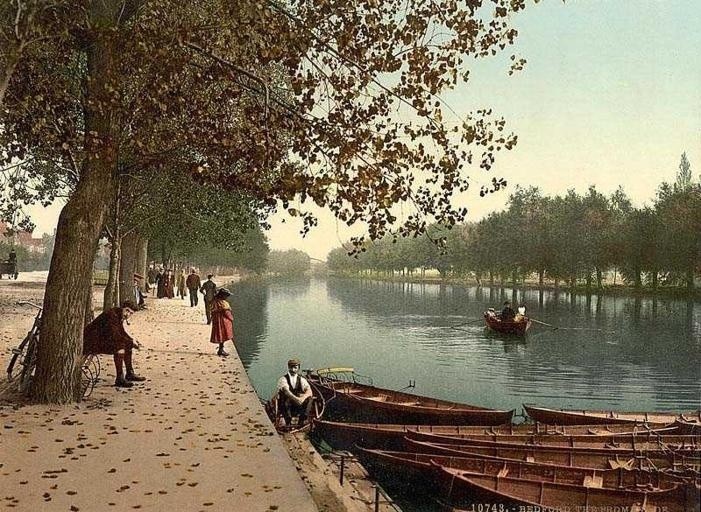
[{"x1": 8, "y1": 301, "x2": 45, "y2": 390}]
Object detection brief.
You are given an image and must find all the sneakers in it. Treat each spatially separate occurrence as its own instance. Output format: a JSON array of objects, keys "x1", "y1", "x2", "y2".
[
  {"x1": 282, "y1": 418, "x2": 306, "y2": 432},
  {"x1": 217, "y1": 350, "x2": 230, "y2": 356}
]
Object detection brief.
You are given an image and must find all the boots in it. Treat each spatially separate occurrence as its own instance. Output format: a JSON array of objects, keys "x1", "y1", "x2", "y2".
[
  {"x1": 124, "y1": 349, "x2": 147, "y2": 381},
  {"x1": 114, "y1": 352, "x2": 134, "y2": 387}
]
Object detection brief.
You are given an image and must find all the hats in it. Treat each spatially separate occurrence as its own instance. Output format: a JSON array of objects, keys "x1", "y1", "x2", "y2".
[
  {"x1": 288, "y1": 359, "x2": 301, "y2": 368},
  {"x1": 217, "y1": 286, "x2": 234, "y2": 296}
]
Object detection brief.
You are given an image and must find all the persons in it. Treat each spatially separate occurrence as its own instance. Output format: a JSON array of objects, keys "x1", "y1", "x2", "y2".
[
  {"x1": 83, "y1": 298, "x2": 148, "y2": 388},
  {"x1": 155, "y1": 268, "x2": 167, "y2": 298},
  {"x1": 209, "y1": 287, "x2": 235, "y2": 357},
  {"x1": 148, "y1": 268, "x2": 156, "y2": 284},
  {"x1": 177, "y1": 269, "x2": 188, "y2": 297},
  {"x1": 187, "y1": 268, "x2": 202, "y2": 307},
  {"x1": 179, "y1": 273, "x2": 186, "y2": 300},
  {"x1": 164, "y1": 270, "x2": 176, "y2": 299},
  {"x1": 200, "y1": 274, "x2": 218, "y2": 325},
  {"x1": 277, "y1": 356, "x2": 314, "y2": 431},
  {"x1": 9, "y1": 248, "x2": 17, "y2": 263},
  {"x1": 502, "y1": 300, "x2": 514, "y2": 322}
]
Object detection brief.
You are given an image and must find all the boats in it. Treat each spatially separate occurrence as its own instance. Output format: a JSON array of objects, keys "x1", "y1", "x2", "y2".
[{"x1": 486, "y1": 310, "x2": 527, "y2": 336}]
